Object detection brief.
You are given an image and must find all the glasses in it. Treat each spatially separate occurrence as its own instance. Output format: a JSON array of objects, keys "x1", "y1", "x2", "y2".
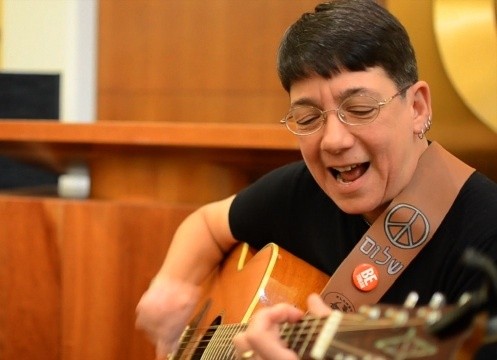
[{"x1": 279, "y1": 80, "x2": 418, "y2": 136}]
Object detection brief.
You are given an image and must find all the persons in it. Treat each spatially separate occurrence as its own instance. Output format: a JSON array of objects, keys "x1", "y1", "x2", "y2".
[{"x1": 135, "y1": 0, "x2": 497, "y2": 360}]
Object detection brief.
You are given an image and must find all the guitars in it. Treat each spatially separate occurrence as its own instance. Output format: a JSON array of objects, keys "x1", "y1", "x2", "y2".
[{"x1": 153, "y1": 241, "x2": 497, "y2": 360}]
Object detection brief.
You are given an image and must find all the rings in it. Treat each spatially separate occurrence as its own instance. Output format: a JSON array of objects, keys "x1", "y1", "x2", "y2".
[{"x1": 241, "y1": 349, "x2": 254, "y2": 358}]
